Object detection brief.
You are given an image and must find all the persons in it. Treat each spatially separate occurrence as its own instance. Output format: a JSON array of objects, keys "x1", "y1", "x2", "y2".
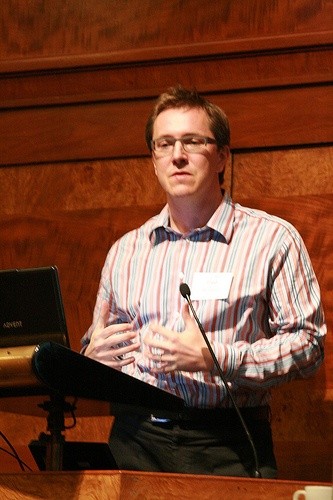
[{"x1": 81, "y1": 86, "x2": 326, "y2": 480}]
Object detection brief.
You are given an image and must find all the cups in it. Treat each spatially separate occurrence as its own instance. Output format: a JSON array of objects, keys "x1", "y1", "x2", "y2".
[{"x1": 292, "y1": 485, "x2": 332, "y2": 500}]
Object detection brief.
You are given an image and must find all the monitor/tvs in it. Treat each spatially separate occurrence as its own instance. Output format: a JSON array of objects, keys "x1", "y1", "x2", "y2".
[{"x1": 1, "y1": 266, "x2": 71, "y2": 350}]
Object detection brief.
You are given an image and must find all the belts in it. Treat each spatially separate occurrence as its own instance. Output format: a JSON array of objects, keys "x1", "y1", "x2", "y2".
[{"x1": 110, "y1": 403, "x2": 271, "y2": 425}]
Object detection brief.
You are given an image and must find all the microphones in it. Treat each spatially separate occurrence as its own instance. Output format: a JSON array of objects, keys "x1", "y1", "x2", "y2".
[{"x1": 180, "y1": 283, "x2": 262, "y2": 478}]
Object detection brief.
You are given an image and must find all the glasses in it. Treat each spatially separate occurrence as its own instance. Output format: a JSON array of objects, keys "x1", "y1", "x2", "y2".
[{"x1": 150, "y1": 135, "x2": 217, "y2": 151}]
